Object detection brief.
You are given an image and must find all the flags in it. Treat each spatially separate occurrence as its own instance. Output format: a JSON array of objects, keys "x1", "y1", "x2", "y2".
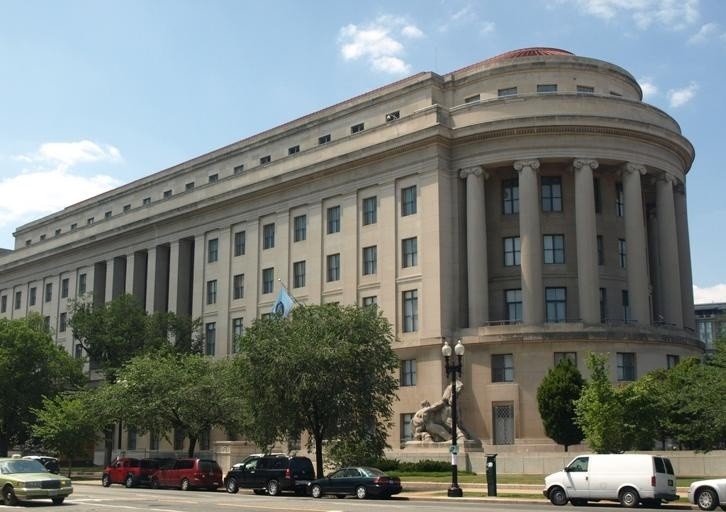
[{"x1": 269, "y1": 282, "x2": 296, "y2": 322}]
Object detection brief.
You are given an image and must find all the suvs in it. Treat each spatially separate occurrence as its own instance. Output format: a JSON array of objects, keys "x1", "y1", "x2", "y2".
[
  {"x1": 23, "y1": 455, "x2": 59, "y2": 475},
  {"x1": 224, "y1": 451, "x2": 315, "y2": 496}
]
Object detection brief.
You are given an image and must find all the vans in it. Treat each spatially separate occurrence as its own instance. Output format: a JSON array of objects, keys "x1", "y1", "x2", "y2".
[
  {"x1": 543, "y1": 454, "x2": 679, "y2": 508},
  {"x1": 101, "y1": 456, "x2": 223, "y2": 491}
]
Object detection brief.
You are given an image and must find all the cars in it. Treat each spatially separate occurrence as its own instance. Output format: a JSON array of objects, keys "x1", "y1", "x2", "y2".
[
  {"x1": 0, "y1": 454, "x2": 73, "y2": 506},
  {"x1": 306, "y1": 467, "x2": 403, "y2": 499},
  {"x1": 688, "y1": 478, "x2": 726, "y2": 511}
]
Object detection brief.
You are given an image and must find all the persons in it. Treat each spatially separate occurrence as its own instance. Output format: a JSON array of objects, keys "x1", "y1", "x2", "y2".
[{"x1": 409, "y1": 397, "x2": 448, "y2": 444}]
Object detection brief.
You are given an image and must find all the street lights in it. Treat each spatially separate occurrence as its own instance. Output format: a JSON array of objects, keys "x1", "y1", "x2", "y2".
[{"x1": 441, "y1": 340, "x2": 465, "y2": 497}]
